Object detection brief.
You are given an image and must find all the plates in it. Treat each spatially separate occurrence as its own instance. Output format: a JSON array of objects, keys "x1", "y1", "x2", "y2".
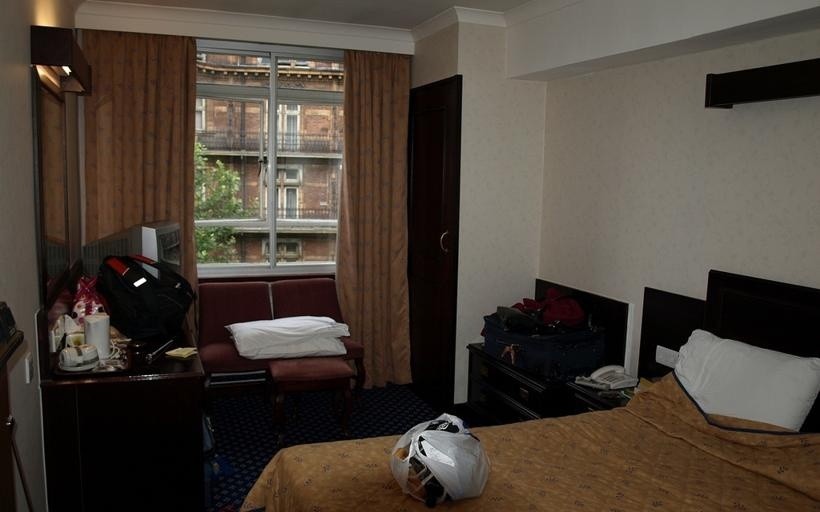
[{"x1": 58, "y1": 363, "x2": 99, "y2": 372}]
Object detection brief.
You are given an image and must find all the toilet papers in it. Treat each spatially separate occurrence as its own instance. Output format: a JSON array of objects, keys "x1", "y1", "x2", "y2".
[{"x1": 85, "y1": 315, "x2": 111, "y2": 361}]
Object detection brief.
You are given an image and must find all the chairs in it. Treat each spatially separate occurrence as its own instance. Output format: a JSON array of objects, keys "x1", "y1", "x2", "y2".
[{"x1": 197, "y1": 277, "x2": 365, "y2": 424}]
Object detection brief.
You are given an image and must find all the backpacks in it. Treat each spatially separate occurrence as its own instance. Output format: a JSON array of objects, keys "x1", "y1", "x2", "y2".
[{"x1": 99, "y1": 255, "x2": 194, "y2": 331}]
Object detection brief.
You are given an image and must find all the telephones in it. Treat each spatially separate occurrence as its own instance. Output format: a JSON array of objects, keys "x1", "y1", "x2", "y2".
[{"x1": 590, "y1": 365, "x2": 639, "y2": 390}]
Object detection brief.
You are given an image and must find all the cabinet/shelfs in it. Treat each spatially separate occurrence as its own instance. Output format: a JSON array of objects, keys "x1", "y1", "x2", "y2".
[{"x1": 465, "y1": 338, "x2": 568, "y2": 426}]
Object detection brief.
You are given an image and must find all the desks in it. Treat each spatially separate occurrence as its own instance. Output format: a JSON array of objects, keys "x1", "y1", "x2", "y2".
[{"x1": 41, "y1": 323, "x2": 205, "y2": 512}]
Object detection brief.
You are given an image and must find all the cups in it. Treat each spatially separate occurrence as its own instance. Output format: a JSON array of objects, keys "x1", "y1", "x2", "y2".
[{"x1": 61, "y1": 334, "x2": 99, "y2": 367}]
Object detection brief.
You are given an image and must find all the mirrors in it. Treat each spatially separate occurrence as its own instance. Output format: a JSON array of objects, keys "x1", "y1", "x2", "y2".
[{"x1": 31, "y1": 32, "x2": 92, "y2": 314}]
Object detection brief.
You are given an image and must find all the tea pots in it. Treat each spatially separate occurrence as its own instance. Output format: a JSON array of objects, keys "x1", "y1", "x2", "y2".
[{"x1": 83, "y1": 312, "x2": 110, "y2": 359}]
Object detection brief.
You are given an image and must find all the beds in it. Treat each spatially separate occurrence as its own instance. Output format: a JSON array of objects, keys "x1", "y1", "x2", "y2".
[{"x1": 239, "y1": 268, "x2": 820, "y2": 511}]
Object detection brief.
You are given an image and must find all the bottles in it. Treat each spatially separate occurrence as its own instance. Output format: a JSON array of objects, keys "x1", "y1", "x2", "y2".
[{"x1": 425, "y1": 480, "x2": 443, "y2": 508}]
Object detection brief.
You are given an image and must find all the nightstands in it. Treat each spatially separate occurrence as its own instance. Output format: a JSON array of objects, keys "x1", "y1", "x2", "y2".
[{"x1": 562, "y1": 378, "x2": 621, "y2": 411}]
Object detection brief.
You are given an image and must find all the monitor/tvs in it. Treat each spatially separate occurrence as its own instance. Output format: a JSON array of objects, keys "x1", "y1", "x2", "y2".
[{"x1": 81, "y1": 220, "x2": 184, "y2": 282}]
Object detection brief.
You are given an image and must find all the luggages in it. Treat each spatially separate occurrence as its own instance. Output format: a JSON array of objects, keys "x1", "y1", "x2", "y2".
[{"x1": 481, "y1": 288, "x2": 606, "y2": 384}]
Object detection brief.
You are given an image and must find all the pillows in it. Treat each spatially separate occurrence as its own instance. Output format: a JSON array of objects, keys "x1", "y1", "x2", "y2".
[{"x1": 670, "y1": 328, "x2": 820, "y2": 439}]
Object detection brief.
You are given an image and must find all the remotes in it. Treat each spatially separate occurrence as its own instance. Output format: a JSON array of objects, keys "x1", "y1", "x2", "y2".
[{"x1": 575, "y1": 378, "x2": 610, "y2": 391}]
